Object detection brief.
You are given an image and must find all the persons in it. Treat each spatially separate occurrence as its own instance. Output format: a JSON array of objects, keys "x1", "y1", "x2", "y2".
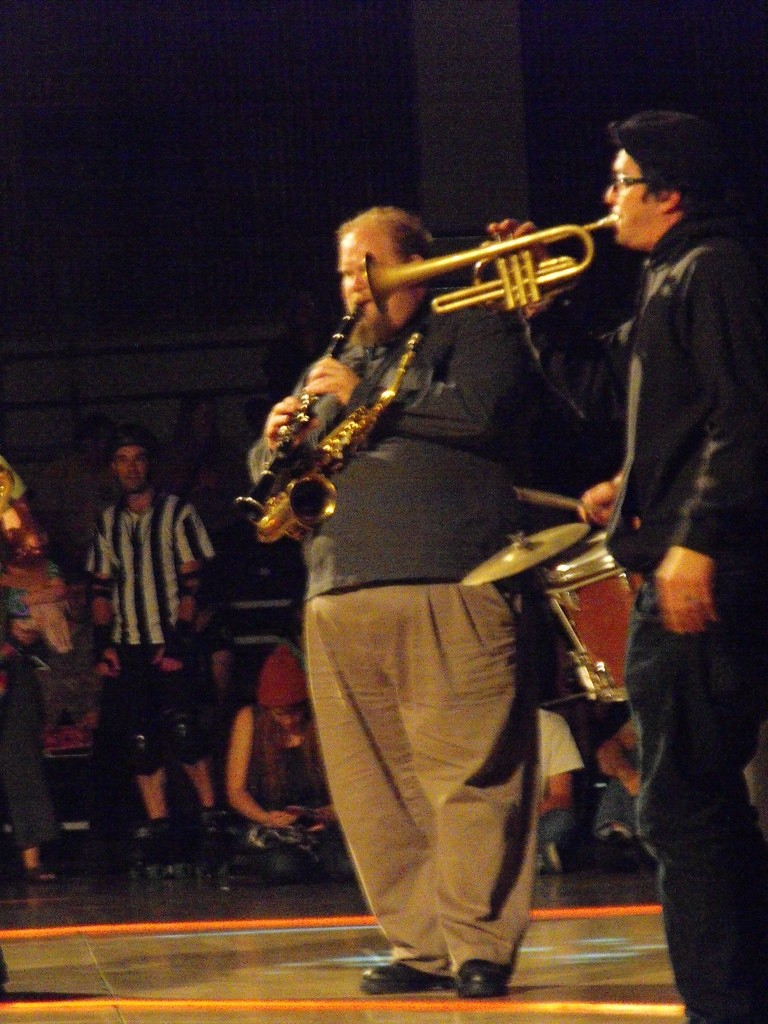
[
  {"x1": 536, "y1": 709, "x2": 643, "y2": 880},
  {"x1": 248, "y1": 205, "x2": 549, "y2": 998},
  {"x1": 2, "y1": 439, "x2": 349, "y2": 895},
  {"x1": 481, "y1": 110, "x2": 768, "y2": 1024}
]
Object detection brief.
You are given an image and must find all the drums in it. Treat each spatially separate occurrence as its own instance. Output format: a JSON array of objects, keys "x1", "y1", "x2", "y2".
[{"x1": 541, "y1": 529, "x2": 647, "y2": 702}]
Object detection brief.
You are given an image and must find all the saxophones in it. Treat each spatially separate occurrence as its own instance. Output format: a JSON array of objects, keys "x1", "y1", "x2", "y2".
[{"x1": 257, "y1": 334, "x2": 421, "y2": 541}]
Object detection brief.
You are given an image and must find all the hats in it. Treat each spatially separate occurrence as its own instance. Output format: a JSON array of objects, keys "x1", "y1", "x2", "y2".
[
  {"x1": 259, "y1": 643, "x2": 309, "y2": 709},
  {"x1": 101, "y1": 423, "x2": 151, "y2": 468},
  {"x1": 617, "y1": 111, "x2": 734, "y2": 197}
]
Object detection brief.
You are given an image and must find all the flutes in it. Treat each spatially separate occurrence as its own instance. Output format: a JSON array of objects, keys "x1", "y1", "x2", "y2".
[{"x1": 234, "y1": 300, "x2": 363, "y2": 519}]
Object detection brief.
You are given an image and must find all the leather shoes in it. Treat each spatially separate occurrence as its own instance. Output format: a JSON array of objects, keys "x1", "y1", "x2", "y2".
[
  {"x1": 360, "y1": 960, "x2": 456, "y2": 994},
  {"x1": 457, "y1": 959, "x2": 507, "y2": 999}
]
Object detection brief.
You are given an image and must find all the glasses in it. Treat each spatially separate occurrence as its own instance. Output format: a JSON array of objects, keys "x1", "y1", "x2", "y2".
[{"x1": 609, "y1": 175, "x2": 646, "y2": 194}]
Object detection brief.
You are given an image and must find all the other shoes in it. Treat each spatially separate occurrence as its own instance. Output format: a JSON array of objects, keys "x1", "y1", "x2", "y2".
[
  {"x1": 203, "y1": 811, "x2": 223, "y2": 833},
  {"x1": 24, "y1": 865, "x2": 59, "y2": 884},
  {"x1": 601, "y1": 830, "x2": 642, "y2": 876},
  {"x1": 545, "y1": 868, "x2": 569, "y2": 884},
  {"x1": 135, "y1": 819, "x2": 172, "y2": 841}
]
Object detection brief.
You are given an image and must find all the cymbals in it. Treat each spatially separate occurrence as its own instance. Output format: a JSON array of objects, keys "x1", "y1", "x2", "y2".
[{"x1": 462, "y1": 524, "x2": 589, "y2": 587}]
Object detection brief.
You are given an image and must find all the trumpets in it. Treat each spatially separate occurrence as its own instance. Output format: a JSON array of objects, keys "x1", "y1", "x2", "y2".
[{"x1": 363, "y1": 216, "x2": 622, "y2": 322}]
